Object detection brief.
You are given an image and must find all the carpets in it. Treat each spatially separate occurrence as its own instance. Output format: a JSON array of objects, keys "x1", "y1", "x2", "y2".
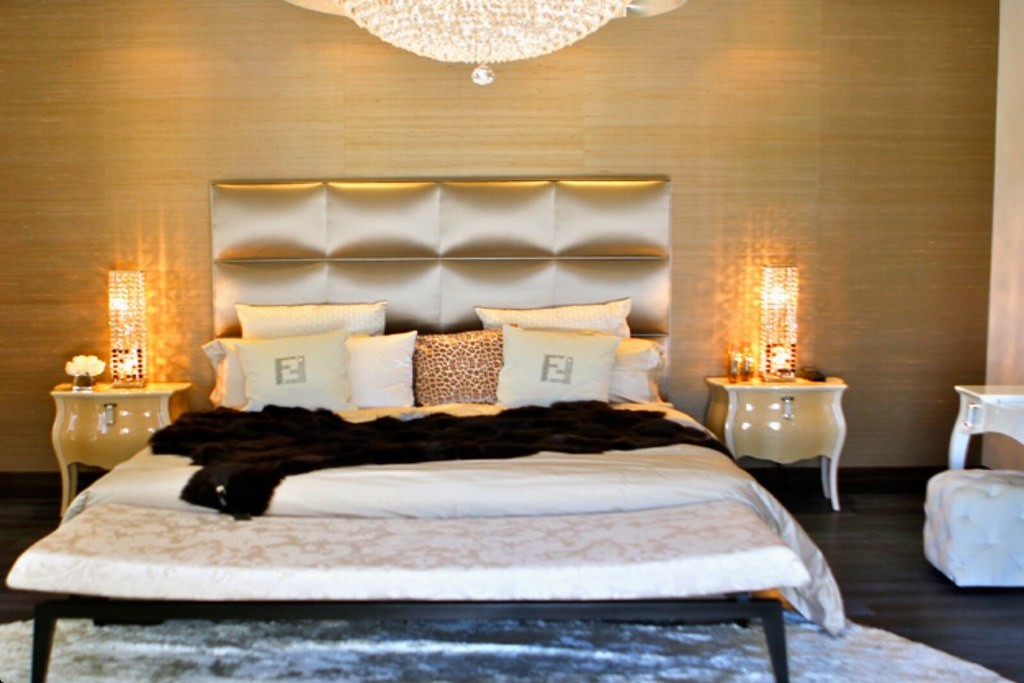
[{"x1": 0, "y1": 619, "x2": 1016, "y2": 683}]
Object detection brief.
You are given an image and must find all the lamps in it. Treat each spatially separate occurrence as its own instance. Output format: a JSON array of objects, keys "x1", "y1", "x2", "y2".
[
  {"x1": 758, "y1": 264, "x2": 799, "y2": 382},
  {"x1": 63, "y1": 354, "x2": 107, "y2": 393},
  {"x1": 282, "y1": 0, "x2": 687, "y2": 88},
  {"x1": 106, "y1": 268, "x2": 149, "y2": 387}
]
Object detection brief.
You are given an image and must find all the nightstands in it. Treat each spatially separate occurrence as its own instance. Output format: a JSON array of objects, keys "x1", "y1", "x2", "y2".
[
  {"x1": 49, "y1": 382, "x2": 192, "y2": 517},
  {"x1": 704, "y1": 375, "x2": 848, "y2": 512}
]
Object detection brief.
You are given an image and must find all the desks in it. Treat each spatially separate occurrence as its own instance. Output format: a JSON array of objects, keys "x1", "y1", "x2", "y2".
[{"x1": 948, "y1": 385, "x2": 1024, "y2": 469}]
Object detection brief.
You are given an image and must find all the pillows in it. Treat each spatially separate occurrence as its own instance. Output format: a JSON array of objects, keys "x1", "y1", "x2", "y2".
[
  {"x1": 413, "y1": 324, "x2": 519, "y2": 406},
  {"x1": 493, "y1": 325, "x2": 620, "y2": 408},
  {"x1": 200, "y1": 328, "x2": 417, "y2": 409},
  {"x1": 611, "y1": 338, "x2": 666, "y2": 403},
  {"x1": 234, "y1": 302, "x2": 386, "y2": 337},
  {"x1": 230, "y1": 331, "x2": 354, "y2": 412},
  {"x1": 474, "y1": 298, "x2": 632, "y2": 338}
]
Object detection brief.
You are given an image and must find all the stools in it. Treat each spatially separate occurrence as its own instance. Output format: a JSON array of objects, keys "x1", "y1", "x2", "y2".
[{"x1": 923, "y1": 469, "x2": 1024, "y2": 587}]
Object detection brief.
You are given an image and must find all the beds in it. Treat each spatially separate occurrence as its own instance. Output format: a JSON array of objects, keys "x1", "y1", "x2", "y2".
[{"x1": 6, "y1": 180, "x2": 845, "y2": 683}]
